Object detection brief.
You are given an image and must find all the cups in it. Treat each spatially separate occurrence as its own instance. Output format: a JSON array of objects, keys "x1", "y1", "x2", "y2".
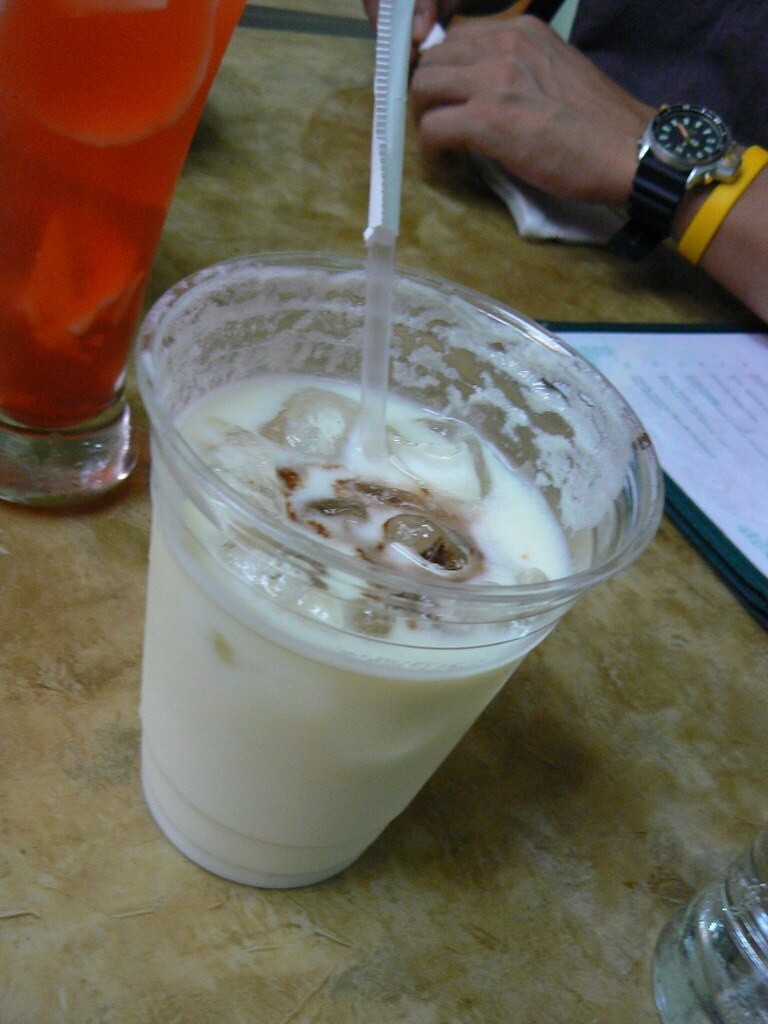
[
  {"x1": 0, "y1": 0, "x2": 247, "y2": 507},
  {"x1": 136, "y1": 251, "x2": 665, "y2": 889}
]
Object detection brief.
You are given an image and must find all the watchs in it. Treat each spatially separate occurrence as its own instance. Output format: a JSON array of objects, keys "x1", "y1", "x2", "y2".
[{"x1": 619, "y1": 103, "x2": 742, "y2": 261}]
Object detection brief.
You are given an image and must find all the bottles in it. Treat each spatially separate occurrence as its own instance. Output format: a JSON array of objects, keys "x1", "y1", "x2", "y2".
[{"x1": 655, "y1": 822, "x2": 768, "y2": 1024}]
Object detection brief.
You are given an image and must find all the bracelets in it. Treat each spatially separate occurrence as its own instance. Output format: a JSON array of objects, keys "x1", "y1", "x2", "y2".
[{"x1": 676, "y1": 144, "x2": 768, "y2": 265}]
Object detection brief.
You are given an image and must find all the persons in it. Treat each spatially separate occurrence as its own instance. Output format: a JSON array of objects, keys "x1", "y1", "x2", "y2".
[{"x1": 362, "y1": 0, "x2": 768, "y2": 336}]
